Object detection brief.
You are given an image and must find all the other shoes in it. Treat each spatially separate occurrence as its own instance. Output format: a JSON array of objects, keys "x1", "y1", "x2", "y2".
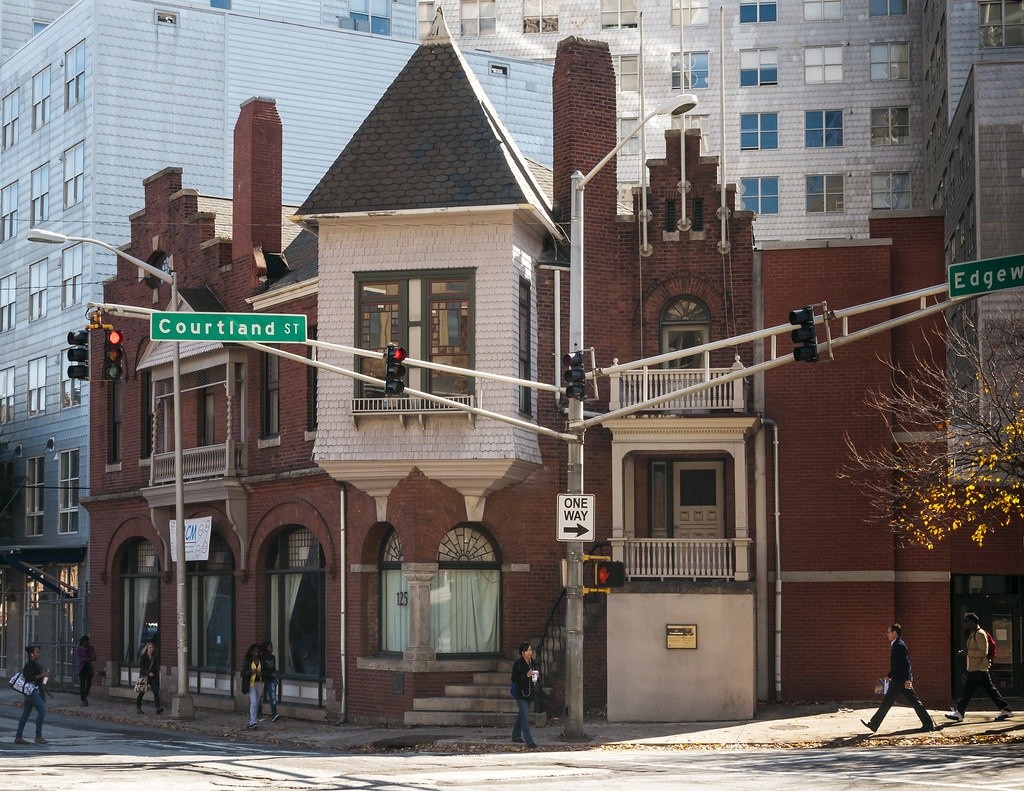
[
  {"x1": 156, "y1": 707, "x2": 164, "y2": 715},
  {"x1": 271, "y1": 713, "x2": 280, "y2": 721},
  {"x1": 82, "y1": 699, "x2": 88, "y2": 706},
  {"x1": 34, "y1": 736, "x2": 49, "y2": 743},
  {"x1": 246, "y1": 723, "x2": 259, "y2": 729},
  {"x1": 861, "y1": 719, "x2": 878, "y2": 732},
  {"x1": 14, "y1": 736, "x2": 28, "y2": 744},
  {"x1": 258, "y1": 717, "x2": 264, "y2": 721},
  {"x1": 914, "y1": 725, "x2": 934, "y2": 732},
  {"x1": 137, "y1": 709, "x2": 144, "y2": 714},
  {"x1": 512, "y1": 739, "x2": 524, "y2": 743},
  {"x1": 528, "y1": 743, "x2": 540, "y2": 749}
]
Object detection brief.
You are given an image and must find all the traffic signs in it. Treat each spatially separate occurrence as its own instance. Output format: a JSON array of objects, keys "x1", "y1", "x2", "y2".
[{"x1": 556, "y1": 492, "x2": 596, "y2": 543}]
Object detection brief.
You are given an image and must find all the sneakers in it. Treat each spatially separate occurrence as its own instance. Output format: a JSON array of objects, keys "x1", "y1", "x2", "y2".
[
  {"x1": 995, "y1": 709, "x2": 1014, "y2": 720},
  {"x1": 944, "y1": 709, "x2": 964, "y2": 722}
]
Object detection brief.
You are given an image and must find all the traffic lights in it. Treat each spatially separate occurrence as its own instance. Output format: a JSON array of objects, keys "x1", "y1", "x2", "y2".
[
  {"x1": 593, "y1": 559, "x2": 626, "y2": 588},
  {"x1": 103, "y1": 328, "x2": 123, "y2": 380},
  {"x1": 384, "y1": 344, "x2": 407, "y2": 395},
  {"x1": 562, "y1": 350, "x2": 587, "y2": 402},
  {"x1": 67, "y1": 328, "x2": 92, "y2": 382},
  {"x1": 789, "y1": 306, "x2": 820, "y2": 364}
]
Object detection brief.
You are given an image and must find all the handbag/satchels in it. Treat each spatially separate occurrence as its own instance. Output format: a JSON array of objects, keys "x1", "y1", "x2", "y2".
[
  {"x1": 242, "y1": 673, "x2": 249, "y2": 694},
  {"x1": 134, "y1": 676, "x2": 148, "y2": 693},
  {"x1": 510, "y1": 661, "x2": 522, "y2": 698},
  {"x1": 9, "y1": 663, "x2": 38, "y2": 696}
]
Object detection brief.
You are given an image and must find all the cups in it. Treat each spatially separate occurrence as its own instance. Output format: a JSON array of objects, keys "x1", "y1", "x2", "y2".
[
  {"x1": 532, "y1": 671, "x2": 538, "y2": 682},
  {"x1": 42, "y1": 677, "x2": 48, "y2": 684}
]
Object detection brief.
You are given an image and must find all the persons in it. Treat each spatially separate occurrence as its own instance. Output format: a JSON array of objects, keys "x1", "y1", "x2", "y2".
[
  {"x1": 861, "y1": 624, "x2": 935, "y2": 734},
  {"x1": 76, "y1": 635, "x2": 96, "y2": 707},
  {"x1": 13, "y1": 646, "x2": 52, "y2": 744},
  {"x1": 136, "y1": 643, "x2": 165, "y2": 715},
  {"x1": 240, "y1": 644, "x2": 266, "y2": 730},
  {"x1": 258, "y1": 641, "x2": 280, "y2": 722},
  {"x1": 510, "y1": 641, "x2": 543, "y2": 749},
  {"x1": 945, "y1": 613, "x2": 1014, "y2": 722}
]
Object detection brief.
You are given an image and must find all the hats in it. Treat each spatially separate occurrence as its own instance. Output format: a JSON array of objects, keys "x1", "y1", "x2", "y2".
[{"x1": 965, "y1": 614, "x2": 979, "y2": 623}]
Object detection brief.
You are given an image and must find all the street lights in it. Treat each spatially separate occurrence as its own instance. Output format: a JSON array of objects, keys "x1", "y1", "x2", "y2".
[
  {"x1": 557, "y1": 93, "x2": 697, "y2": 740},
  {"x1": 24, "y1": 227, "x2": 197, "y2": 722}
]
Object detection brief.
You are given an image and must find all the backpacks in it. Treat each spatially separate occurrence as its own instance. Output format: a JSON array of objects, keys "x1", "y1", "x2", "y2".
[{"x1": 974, "y1": 628, "x2": 996, "y2": 660}]
